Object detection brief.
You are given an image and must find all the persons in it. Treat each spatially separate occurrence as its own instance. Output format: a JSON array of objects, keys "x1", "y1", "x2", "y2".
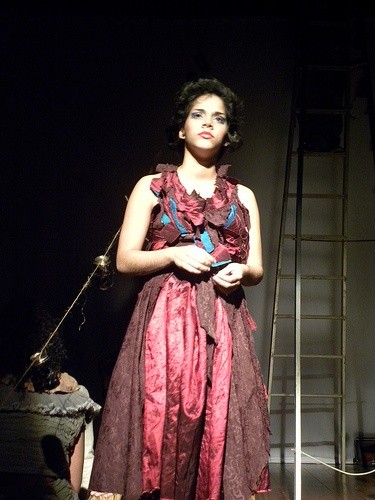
[
  {"x1": 0, "y1": 304, "x2": 101, "y2": 500},
  {"x1": 87, "y1": 79, "x2": 271, "y2": 500}
]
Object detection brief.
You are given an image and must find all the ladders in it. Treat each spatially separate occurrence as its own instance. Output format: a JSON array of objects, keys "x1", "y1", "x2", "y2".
[{"x1": 258, "y1": 48, "x2": 352, "y2": 449}]
